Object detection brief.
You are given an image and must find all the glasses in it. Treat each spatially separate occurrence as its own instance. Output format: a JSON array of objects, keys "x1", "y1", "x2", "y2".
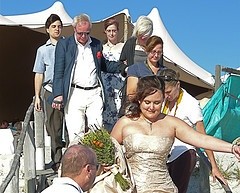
[
  {"x1": 87, "y1": 162, "x2": 99, "y2": 170},
  {"x1": 162, "y1": 75, "x2": 177, "y2": 81}
]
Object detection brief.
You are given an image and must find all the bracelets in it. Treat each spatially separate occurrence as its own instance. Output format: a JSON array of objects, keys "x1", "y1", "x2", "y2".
[{"x1": 231, "y1": 145, "x2": 235, "y2": 153}]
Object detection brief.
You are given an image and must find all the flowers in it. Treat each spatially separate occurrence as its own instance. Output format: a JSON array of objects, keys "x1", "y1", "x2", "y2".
[
  {"x1": 74, "y1": 118, "x2": 132, "y2": 191},
  {"x1": 97, "y1": 51, "x2": 102, "y2": 59}
]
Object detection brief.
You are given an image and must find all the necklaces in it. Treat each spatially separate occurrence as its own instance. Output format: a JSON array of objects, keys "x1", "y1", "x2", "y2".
[{"x1": 145, "y1": 119, "x2": 152, "y2": 130}]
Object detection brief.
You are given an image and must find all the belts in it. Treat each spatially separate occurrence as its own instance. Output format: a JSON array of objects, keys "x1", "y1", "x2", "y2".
[{"x1": 71, "y1": 84, "x2": 100, "y2": 90}]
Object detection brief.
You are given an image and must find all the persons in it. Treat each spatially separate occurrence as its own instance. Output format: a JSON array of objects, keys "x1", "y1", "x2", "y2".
[
  {"x1": 91, "y1": 75, "x2": 240, "y2": 193},
  {"x1": 99, "y1": 20, "x2": 127, "y2": 132},
  {"x1": 159, "y1": 69, "x2": 228, "y2": 193},
  {"x1": 41, "y1": 144, "x2": 96, "y2": 193},
  {"x1": 51, "y1": 14, "x2": 126, "y2": 147},
  {"x1": 33, "y1": 14, "x2": 62, "y2": 171},
  {"x1": 119, "y1": 15, "x2": 153, "y2": 76},
  {"x1": 127, "y1": 36, "x2": 165, "y2": 100}
]
